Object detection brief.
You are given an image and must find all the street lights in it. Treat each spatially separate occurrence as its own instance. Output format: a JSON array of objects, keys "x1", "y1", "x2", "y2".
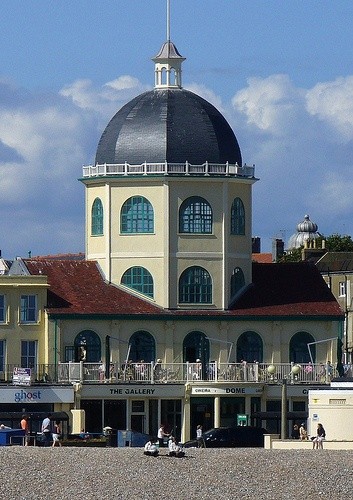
[{"x1": 268, "y1": 364, "x2": 301, "y2": 439}]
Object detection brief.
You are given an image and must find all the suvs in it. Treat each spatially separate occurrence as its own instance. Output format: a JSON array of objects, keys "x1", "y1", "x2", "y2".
[{"x1": 182, "y1": 426, "x2": 270, "y2": 448}]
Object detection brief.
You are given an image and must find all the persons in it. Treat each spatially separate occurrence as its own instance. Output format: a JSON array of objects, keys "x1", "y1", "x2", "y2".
[
  {"x1": 294, "y1": 423, "x2": 306, "y2": 440},
  {"x1": 317, "y1": 361, "x2": 332, "y2": 381},
  {"x1": 197, "y1": 425, "x2": 203, "y2": 448},
  {"x1": 195, "y1": 359, "x2": 215, "y2": 380},
  {"x1": 168, "y1": 433, "x2": 184, "y2": 458},
  {"x1": 158, "y1": 425, "x2": 169, "y2": 446},
  {"x1": 21, "y1": 415, "x2": 29, "y2": 446},
  {"x1": 52, "y1": 420, "x2": 62, "y2": 447},
  {"x1": 313, "y1": 423, "x2": 325, "y2": 449},
  {"x1": 304, "y1": 362, "x2": 312, "y2": 374},
  {"x1": 79, "y1": 429, "x2": 91, "y2": 439},
  {"x1": 144, "y1": 438, "x2": 159, "y2": 456},
  {"x1": 122, "y1": 360, "x2": 146, "y2": 380},
  {"x1": 230, "y1": 359, "x2": 258, "y2": 381},
  {"x1": 154, "y1": 359, "x2": 162, "y2": 380},
  {"x1": 42, "y1": 413, "x2": 53, "y2": 446},
  {"x1": 98, "y1": 362, "x2": 114, "y2": 380}
]
